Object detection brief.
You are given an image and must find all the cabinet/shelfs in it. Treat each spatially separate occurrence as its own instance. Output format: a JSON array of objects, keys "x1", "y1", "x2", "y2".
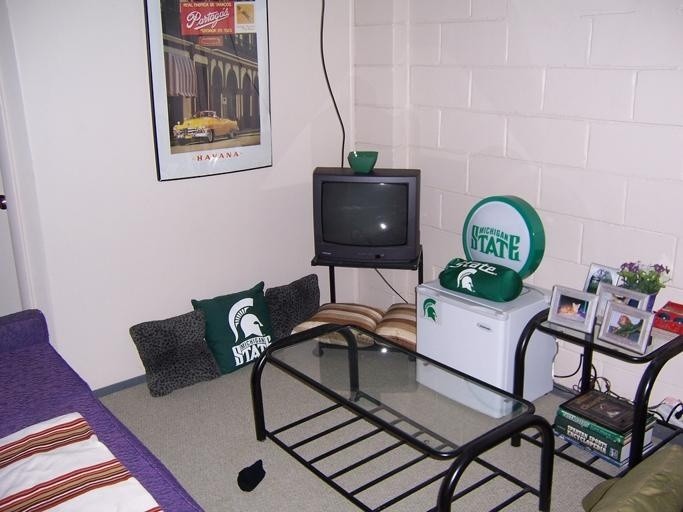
[{"x1": 510, "y1": 309, "x2": 682, "y2": 479}]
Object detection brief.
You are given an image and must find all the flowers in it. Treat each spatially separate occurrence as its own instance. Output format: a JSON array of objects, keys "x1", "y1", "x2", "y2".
[{"x1": 617, "y1": 262, "x2": 671, "y2": 293}]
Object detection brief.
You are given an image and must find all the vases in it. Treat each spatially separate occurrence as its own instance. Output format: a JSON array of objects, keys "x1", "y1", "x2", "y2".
[{"x1": 619, "y1": 285, "x2": 659, "y2": 312}]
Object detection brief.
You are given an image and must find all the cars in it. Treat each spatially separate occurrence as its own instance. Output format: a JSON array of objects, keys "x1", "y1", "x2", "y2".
[{"x1": 173, "y1": 111, "x2": 240, "y2": 142}]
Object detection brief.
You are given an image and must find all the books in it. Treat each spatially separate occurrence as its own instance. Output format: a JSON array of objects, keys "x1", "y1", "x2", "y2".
[{"x1": 553, "y1": 389, "x2": 657, "y2": 470}]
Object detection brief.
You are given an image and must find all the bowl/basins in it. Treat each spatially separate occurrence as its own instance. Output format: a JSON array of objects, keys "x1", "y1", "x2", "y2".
[{"x1": 347, "y1": 150, "x2": 378, "y2": 173}]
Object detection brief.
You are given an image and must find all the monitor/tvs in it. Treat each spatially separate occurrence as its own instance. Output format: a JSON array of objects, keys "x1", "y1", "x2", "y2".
[{"x1": 313, "y1": 166, "x2": 420, "y2": 264}]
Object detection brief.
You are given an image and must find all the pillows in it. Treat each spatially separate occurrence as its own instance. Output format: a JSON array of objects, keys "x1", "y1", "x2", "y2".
[
  {"x1": 130, "y1": 309, "x2": 220, "y2": 398},
  {"x1": 191, "y1": 282, "x2": 275, "y2": 373},
  {"x1": 438, "y1": 257, "x2": 523, "y2": 302},
  {"x1": 293, "y1": 301, "x2": 382, "y2": 349},
  {"x1": 373, "y1": 302, "x2": 417, "y2": 358},
  {"x1": 266, "y1": 274, "x2": 318, "y2": 343}
]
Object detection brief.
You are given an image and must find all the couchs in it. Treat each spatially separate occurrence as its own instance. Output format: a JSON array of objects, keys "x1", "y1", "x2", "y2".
[{"x1": 0, "y1": 309, "x2": 206, "y2": 512}]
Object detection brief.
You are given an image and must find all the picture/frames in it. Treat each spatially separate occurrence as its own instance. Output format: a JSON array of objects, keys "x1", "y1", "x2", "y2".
[
  {"x1": 547, "y1": 262, "x2": 656, "y2": 355},
  {"x1": 144, "y1": 0, "x2": 273, "y2": 182}
]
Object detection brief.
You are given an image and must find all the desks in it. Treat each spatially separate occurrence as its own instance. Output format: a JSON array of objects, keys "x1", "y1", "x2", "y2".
[{"x1": 313, "y1": 245, "x2": 423, "y2": 359}]
